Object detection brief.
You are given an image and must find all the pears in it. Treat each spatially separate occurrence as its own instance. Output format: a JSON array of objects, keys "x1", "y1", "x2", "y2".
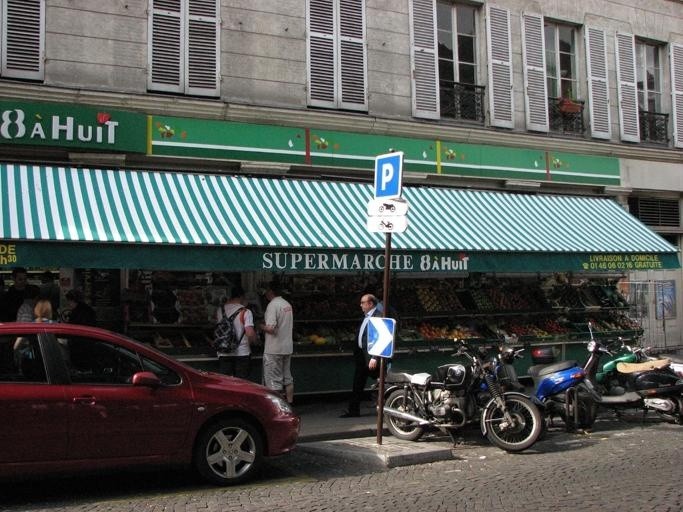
[{"x1": 417, "y1": 288, "x2": 442, "y2": 312}]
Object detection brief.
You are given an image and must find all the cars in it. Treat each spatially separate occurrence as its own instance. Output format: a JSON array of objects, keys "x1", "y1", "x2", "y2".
[{"x1": 0, "y1": 320, "x2": 300, "y2": 487}]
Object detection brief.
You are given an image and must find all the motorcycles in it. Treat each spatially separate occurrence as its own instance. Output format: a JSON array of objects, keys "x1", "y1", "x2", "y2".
[
  {"x1": 371, "y1": 338, "x2": 547, "y2": 452},
  {"x1": 466, "y1": 320, "x2": 683, "y2": 436}
]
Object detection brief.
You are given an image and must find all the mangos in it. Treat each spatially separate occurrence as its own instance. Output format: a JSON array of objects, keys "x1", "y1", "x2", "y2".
[{"x1": 309, "y1": 335, "x2": 327, "y2": 344}]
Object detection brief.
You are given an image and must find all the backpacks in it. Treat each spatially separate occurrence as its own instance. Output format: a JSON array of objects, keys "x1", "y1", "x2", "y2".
[{"x1": 212, "y1": 304, "x2": 249, "y2": 354}]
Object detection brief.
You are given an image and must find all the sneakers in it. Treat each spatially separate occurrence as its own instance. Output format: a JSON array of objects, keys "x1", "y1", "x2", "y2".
[{"x1": 337, "y1": 409, "x2": 361, "y2": 417}]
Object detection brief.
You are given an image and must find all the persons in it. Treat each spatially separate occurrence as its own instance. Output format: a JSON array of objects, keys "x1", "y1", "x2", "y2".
[
  {"x1": 12, "y1": 298, "x2": 57, "y2": 378},
  {"x1": 4, "y1": 267, "x2": 39, "y2": 323},
  {"x1": 255, "y1": 280, "x2": 298, "y2": 412},
  {"x1": 63, "y1": 289, "x2": 97, "y2": 369},
  {"x1": 211, "y1": 287, "x2": 258, "y2": 380},
  {"x1": 37, "y1": 269, "x2": 59, "y2": 322},
  {"x1": 15, "y1": 284, "x2": 41, "y2": 377},
  {"x1": 336, "y1": 292, "x2": 392, "y2": 420}
]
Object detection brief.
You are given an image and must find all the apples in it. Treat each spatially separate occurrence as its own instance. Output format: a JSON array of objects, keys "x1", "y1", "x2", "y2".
[
  {"x1": 420, "y1": 323, "x2": 449, "y2": 340},
  {"x1": 471, "y1": 286, "x2": 530, "y2": 311},
  {"x1": 97, "y1": 110, "x2": 111, "y2": 124}
]
